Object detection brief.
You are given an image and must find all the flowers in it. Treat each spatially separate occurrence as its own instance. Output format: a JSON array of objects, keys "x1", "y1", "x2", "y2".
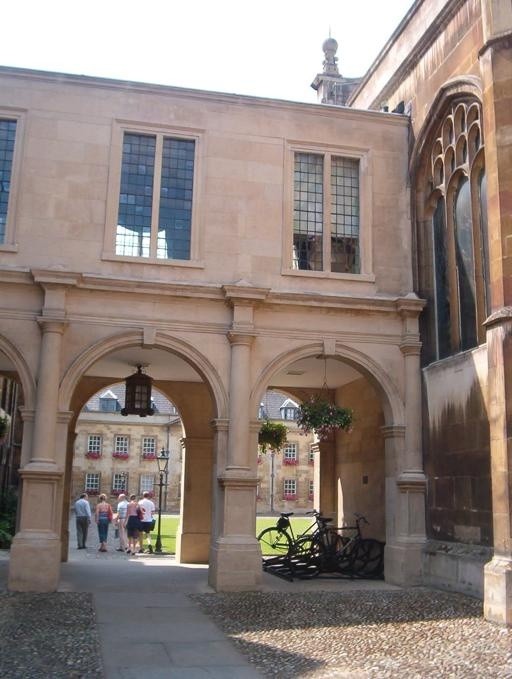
[
  {"x1": 258, "y1": 420, "x2": 289, "y2": 458},
  {"x1": 255, "y1": 492, "x2": 314, "y2": 503},
  {"x1": 257, "y1": 458, "x2": 314, "y2": 467},
  {"x1": 81, "y1": 488, "x2": 156, "y2": 498},
  {"x1": 294, "y1": 392, "x2": 359, "y2": 442},
  {"x1": 84, "y1": 451, "x2": 157, "y2": 461}
]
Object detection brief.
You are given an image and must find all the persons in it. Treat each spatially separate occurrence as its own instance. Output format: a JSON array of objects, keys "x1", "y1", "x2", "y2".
[
  {"x1": 122, "y1": 495, "x2": 145, "y2": 554},
  {"x1": 136, "y1": 490, "x2": 156, "y2": 554},
  {"x1": 114, "y1": 494, "x2": 131, "y2": 552},
  {"x1": 73, "y1": 492, "x2": 92, "y2": 550},
  {"x1": 94, "y1": 493, "x2": 114, "y2": 554}
]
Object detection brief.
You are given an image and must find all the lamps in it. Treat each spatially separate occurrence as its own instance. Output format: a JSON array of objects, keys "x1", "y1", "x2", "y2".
[{"x1": 120, "y1": 363, "x2": 154, "y2": 418}]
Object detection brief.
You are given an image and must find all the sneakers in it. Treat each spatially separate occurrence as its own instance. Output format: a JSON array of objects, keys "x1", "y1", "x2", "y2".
[{"x1": 117, "y1": 545, "x2": 153, "y2": 555}]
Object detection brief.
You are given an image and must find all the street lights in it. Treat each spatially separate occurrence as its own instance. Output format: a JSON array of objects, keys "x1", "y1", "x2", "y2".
[
  {"x1": 151, "y1": 448, "x2": 170, "y2": 553},
  {"x1": 268, "y1": 445, "x2": 276, "y2": 513}
]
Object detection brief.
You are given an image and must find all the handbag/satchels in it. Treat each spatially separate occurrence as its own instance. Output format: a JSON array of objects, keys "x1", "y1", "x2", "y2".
[
  {"x1": 108, "y1": 510, "x2": 112, "y2": 520},
  {"x1": 136, "y1": 506, "x2": 143, "y2": 520}
]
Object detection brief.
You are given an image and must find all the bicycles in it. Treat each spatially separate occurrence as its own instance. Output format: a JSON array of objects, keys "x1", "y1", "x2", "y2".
[{"x1": 258, "y1": 508, "x2": 384, "y2": 579}]
